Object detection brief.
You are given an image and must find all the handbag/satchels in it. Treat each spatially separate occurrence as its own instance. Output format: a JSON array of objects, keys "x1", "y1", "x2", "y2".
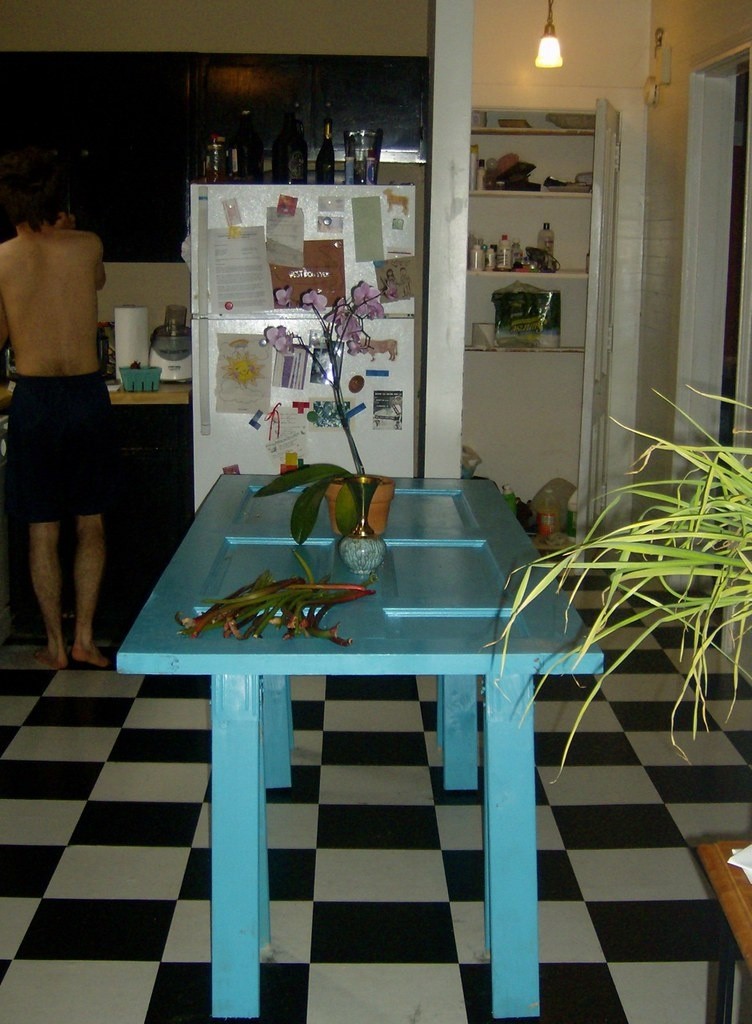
[{"x1": 491, "y1": 280, "x2": 562, "y2": 348}]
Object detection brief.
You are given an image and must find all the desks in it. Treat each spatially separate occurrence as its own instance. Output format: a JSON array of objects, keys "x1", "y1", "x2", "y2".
[
  {"x1": 0, "y1": 375, "x2": 192, "y2": 410},
  {"x1": 115, "y1": 475, "x2": 605, "y2": 1019},
  {"x1": 697, "y1": 842, "x2": 751, "y2": 1024}
]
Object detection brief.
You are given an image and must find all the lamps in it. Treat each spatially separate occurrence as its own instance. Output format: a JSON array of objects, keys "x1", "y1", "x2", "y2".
[{"x1": 535, "y1": 0, "x2": 564, "y2": 69}]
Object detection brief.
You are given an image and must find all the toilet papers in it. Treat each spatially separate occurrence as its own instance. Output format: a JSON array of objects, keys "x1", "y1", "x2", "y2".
[{"x1": 114, "y1": 305, "x2": 149, "y2": 382}]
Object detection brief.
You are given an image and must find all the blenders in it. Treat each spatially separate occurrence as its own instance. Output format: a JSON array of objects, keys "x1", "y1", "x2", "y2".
[{"x1": 148, "y1": 305, "x2": 192, "y2": 384}]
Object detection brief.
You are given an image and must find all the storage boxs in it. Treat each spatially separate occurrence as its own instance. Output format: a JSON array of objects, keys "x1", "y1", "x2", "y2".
[{"x1": 120, "y1": 367, "x2": 161, "y2": 392}]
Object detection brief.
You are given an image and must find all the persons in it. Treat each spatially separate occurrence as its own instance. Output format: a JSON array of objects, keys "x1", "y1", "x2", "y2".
[{"x1": 0, "y1": 178, "x2": 109, "y2": 668}]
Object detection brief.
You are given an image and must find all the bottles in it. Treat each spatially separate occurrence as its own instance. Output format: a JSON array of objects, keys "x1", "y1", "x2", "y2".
[
  {"x1": 314, "y1": 117, "x2": 335, "y2": 184},
  {"x1": 203, "y1": 134, "x2": 226, "y2": 184},
  {"x1": 536, "y1": 487, "x2": 561, "y2": 537},
  {"x1": 225, "y1": 104, "x2": 264, "y2": 184},
  {"x1": 272, "y1": 112, "x2": 307, "y2": 185},
  {"x1": 511, "y1": 237, "x2": 523, "y2": 265},
  {"x1": 469, "y1": 240, "x2": 485, "y2": 272},
  {"x1": 502, "y1": 484, "x2": 517, "y2": 517},
  {"x1": 496, "y1": 234, "x2": 513, "y2": 271},
  {"x1": 476, "y1": 159, "x2": 486, "y2": 191},
  {"x1": 485, "y1": 249, "x2": 496, "y2": 270},
  {"x1": 537, "y1": 223, "x2": 554, "y2": 256}
]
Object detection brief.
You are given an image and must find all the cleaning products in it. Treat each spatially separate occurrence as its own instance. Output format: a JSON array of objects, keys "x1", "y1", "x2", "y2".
[
  {"x1": 469, "y1": 143, "x2": 486, "y2": 191},
  {"x1": 502, "y1": 484, "x2": 578, "y2": 543}
]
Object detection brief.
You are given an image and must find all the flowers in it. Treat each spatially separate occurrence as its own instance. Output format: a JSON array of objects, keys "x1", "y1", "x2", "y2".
[{"x1": 252, "y1": 282, "x2": 401, "y2": 545}]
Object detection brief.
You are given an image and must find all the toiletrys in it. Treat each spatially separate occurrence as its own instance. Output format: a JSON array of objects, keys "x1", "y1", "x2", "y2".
[{"x1": 469, "y1": 222, "x2": 554, "y2": 273}]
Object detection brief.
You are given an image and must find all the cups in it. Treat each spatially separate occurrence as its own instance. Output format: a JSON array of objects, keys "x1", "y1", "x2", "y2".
[{"x1": 343, "y1": 127, "x2": 383, "y2": 185}]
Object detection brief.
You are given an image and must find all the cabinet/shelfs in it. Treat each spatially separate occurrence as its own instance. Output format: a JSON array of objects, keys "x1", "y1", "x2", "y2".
[
  {"x1": 465, "y1": 127, "x2": 595, "y2": 353},
  {"x1": 0, "y1": 51, "x2": 196, "y2": 261},
  {"x1": 195, "y1": 53, "x2": 428, "y2": 164}
]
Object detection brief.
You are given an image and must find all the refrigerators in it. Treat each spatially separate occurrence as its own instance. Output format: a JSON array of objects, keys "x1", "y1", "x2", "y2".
[{"x1": 189, "y1": 184, "x2": 423, "y2": 520}]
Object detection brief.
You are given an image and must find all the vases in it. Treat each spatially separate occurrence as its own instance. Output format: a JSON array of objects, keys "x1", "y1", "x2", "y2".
[
  {"x1": 338, "y1": 477, "x2": 386, "y2": 574},
  {"x1": 326, "y1": 479, "x2": 396, "y2": 536}
]
